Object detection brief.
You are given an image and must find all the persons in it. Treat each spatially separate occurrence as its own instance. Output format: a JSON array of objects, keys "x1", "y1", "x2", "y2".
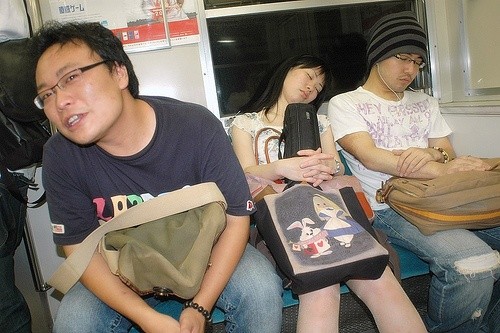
[
  {"x1": 325, "y1": 11, "x2": 500, "y2": 333},
  {"x1": 29, "y1": 18, "x2": 284, "y2": 333},
  {"x1": 231, "y1": 52, "x2": 427, "y2": 333}
]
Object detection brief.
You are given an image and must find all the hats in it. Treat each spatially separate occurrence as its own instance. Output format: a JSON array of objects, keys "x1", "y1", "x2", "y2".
[{"x1": 366, "y1": 11, "x2": 428, "y2": 71}]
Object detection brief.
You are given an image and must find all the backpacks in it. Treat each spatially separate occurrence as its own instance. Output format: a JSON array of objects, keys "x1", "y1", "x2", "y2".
[{"x1": 0, "y1": 38, "x2": 57, "y2": 208}]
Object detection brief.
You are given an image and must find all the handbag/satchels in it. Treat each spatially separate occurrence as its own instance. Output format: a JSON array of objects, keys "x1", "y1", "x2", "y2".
[
  {"x1": 375, "y1": 158, "x2": 500, "y2": 236},
  {"x1": 250, "y1": 126, "x2": 390, "y2": 296},
  {"x1": 0, "y1": 169, "x2": 41, "y2": 258},
  {"x1": 47, "y1": 181, "x2": 228, "y2": 300}
]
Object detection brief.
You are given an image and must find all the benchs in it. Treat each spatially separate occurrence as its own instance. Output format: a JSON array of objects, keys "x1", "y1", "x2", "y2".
[{"x1": 130, "y1": 152, "x2": 433, "y2": 333}]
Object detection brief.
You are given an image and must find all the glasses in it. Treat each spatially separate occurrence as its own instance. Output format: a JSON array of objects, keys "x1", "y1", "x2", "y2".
[
  {"x1": 33, "y1": 60, "x2": 106, "y2": 109},
  {"x1": 395, "y1": 54, "x2": 427, "y2": 69}
]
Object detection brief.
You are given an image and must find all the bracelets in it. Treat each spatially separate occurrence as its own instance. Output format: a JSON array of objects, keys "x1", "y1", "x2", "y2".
[
  {"x1": 428, "y1": 147, "x2": 449, "y2": 163},
  {"x1": 331, "y1": 157, "x2": 341, "y2": 174},
  {"x1": 182, "y1": 302, "x2": 212, "y2": 327}
]
